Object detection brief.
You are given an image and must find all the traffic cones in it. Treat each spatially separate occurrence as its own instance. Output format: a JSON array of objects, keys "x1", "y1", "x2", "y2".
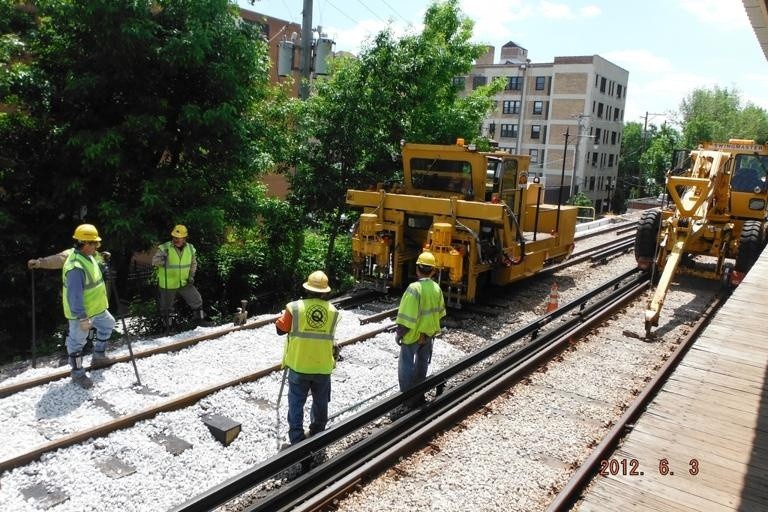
[{"x1": 545, "y1": 281, "x2": 559, "y2": 312}]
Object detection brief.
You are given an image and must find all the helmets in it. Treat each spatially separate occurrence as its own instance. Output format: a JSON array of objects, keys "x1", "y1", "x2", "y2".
[
  {"x1": 303, "y1": 270, "x2": 332, "y2": 293},
  {"x1": 416, "y1": 252, "x2": 437, "y2": 268},
  {"x1": 73, "y1": 224, "x2": 102, "y2": 242},
  {"x1": 171, "y1": 225, "x2": 188, "y2": 238}
]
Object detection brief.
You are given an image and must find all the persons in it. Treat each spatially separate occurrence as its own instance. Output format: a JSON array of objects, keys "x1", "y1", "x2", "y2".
[
  {"x1": 152, "y1": 223, "x2": 213, "y2": 337},
  {"x1": 61, "y1": 224, "x2": 116, "y2": 390},
  {"x1": 448, "y1": 168, "x2": 467, "y2": 194},
  {"x1": 28, "y1": 240, "x2": 111, "y2": 357},
  {"x1": 274, "y1": 268, "x2": 341, "y2": 446},
  {"x1": 394, "y1": 251, "x2": 447, "y2": 393}
]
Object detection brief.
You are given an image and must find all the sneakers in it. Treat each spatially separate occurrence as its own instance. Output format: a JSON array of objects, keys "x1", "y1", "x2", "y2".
[
  {"x1": 91, "y1": 358, "x2": 116, "y2": 368},
  {"x1": 194, "y1": 319, "x2": 217, "y2": 327},
  {"x1": 72, "y1": 375, "x2": 93, "y2": 389}
]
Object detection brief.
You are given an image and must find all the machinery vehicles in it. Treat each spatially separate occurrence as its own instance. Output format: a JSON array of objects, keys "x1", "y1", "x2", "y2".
[
  {"x1": 634, "y1": 138, "x2": 768, "y2": 337},
  {"x1": 344, "y1": 136, "x2": 579, "y2": 310}
]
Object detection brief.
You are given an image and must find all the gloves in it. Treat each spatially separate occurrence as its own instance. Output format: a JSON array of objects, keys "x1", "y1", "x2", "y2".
[
  {"x1": 189, "y1": 277, "x2": 195, "y2": 285},
  {"x1": 396, "y1": 334, "x2": 402, "y2": 346},
  {"x1": 28, "y1": 259, "x2": 40, "y2": 269}
]
